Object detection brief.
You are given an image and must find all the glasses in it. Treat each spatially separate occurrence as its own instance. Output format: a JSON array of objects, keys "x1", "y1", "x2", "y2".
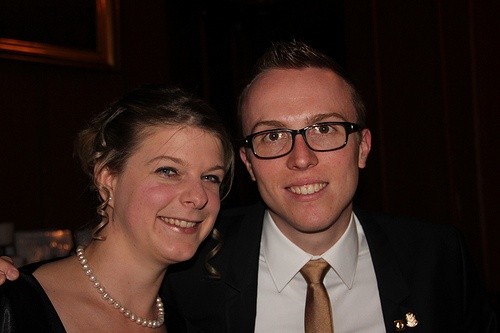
[{"x1": 243, "y1": 121, "x2": 358, "y2": 158}]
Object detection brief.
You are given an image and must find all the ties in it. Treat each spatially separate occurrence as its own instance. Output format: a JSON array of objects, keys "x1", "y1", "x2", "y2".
[{"x1": 299, "y1": 259, "x2": 334, "y2": 332}]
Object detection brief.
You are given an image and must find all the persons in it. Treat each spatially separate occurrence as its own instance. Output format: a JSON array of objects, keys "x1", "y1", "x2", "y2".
[
  {"x1": 0, "y1": 89, "x2": 234, "y2": 333},
  {"x1": 0, "y1": 40, "x2": 500, "y2": 333}
]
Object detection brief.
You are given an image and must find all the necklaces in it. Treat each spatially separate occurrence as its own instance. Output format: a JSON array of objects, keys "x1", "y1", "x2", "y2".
[{"x1": 76, "y1": 244, "x2": 165, "y2": 329}]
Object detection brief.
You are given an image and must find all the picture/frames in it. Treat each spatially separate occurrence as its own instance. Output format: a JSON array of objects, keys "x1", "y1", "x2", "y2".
[{"x1": 0, "y1": 0, "x2": 121, "y2": 73}]
